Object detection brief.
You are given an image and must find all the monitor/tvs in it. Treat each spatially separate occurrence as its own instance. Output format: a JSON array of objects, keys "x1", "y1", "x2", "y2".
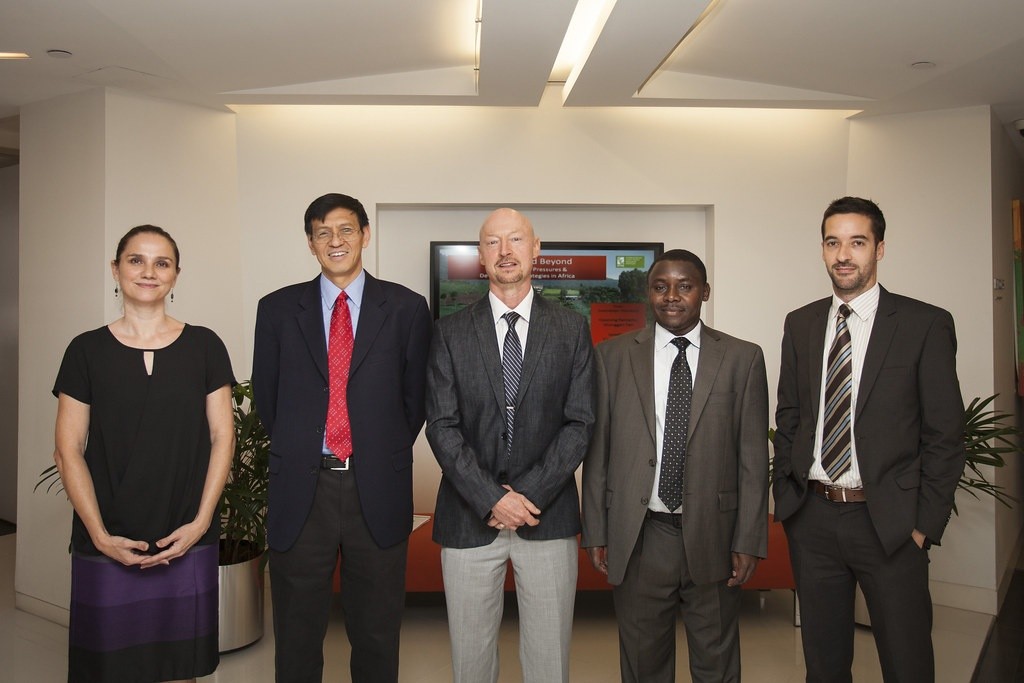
[{"x1": 429, "y1": 240, "x2": 665, "y2": 346}]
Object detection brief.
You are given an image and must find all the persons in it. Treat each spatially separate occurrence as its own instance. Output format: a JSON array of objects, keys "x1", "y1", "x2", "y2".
[
  {"x1": 582, "y1": 250, "x2": 769, "y2": 683},
  {"x1": 423, "y1": 208, "x2": 596, "y2": 683},
  {"x1": 772, "y1": 196, "x2": 966, "y2": 683},
  {"x1": 250, "y1": 192, "x2": 431, "y2": 683},
  {"x1": 53, "y1": 225, "x2": 236, "y2": 683}
]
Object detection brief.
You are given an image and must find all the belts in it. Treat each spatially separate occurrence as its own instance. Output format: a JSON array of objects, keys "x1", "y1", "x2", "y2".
[
  {"x1": 808, "y1": 480, "x2": 865, "y2": 503},
  {"x1": 645, "y1": 509, "x2": 683, "y2": 528},
  {"x1": 321, "y1": 455, "x2": 353, "y2": 471}
]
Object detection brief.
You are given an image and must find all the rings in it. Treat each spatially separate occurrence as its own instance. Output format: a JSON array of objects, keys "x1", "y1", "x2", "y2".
[{"x1": 499, "y1": 522, "x2": 505, "y2": 528}]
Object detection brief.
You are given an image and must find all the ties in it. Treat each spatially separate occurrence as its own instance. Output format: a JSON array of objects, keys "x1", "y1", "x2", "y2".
[
  {"x1": 657, "y1": 337, "x2": 693, "y2": 514},
  {"x1": 325, "y1": 290, "x2": 353, "y2": 463},
  {"x1": 821, "y1": 303, "x2": 852, "y2": 484},
  {"x1": 501, "y1": 312, "x2": 524, "y2": 462}
]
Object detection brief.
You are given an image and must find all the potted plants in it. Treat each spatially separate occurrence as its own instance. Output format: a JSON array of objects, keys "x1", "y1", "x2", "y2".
[
  {"x1": 769, "y1": 392, "x2": 1024, "y2": 627},
  {"x1": 31, "y1": 373, "x2": 272, "y2": 657}
]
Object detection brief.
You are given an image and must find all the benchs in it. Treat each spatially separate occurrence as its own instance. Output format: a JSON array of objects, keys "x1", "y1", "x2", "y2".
[{"x1": 324, "y1": 512, "x2": 802, "y2": 627}]
